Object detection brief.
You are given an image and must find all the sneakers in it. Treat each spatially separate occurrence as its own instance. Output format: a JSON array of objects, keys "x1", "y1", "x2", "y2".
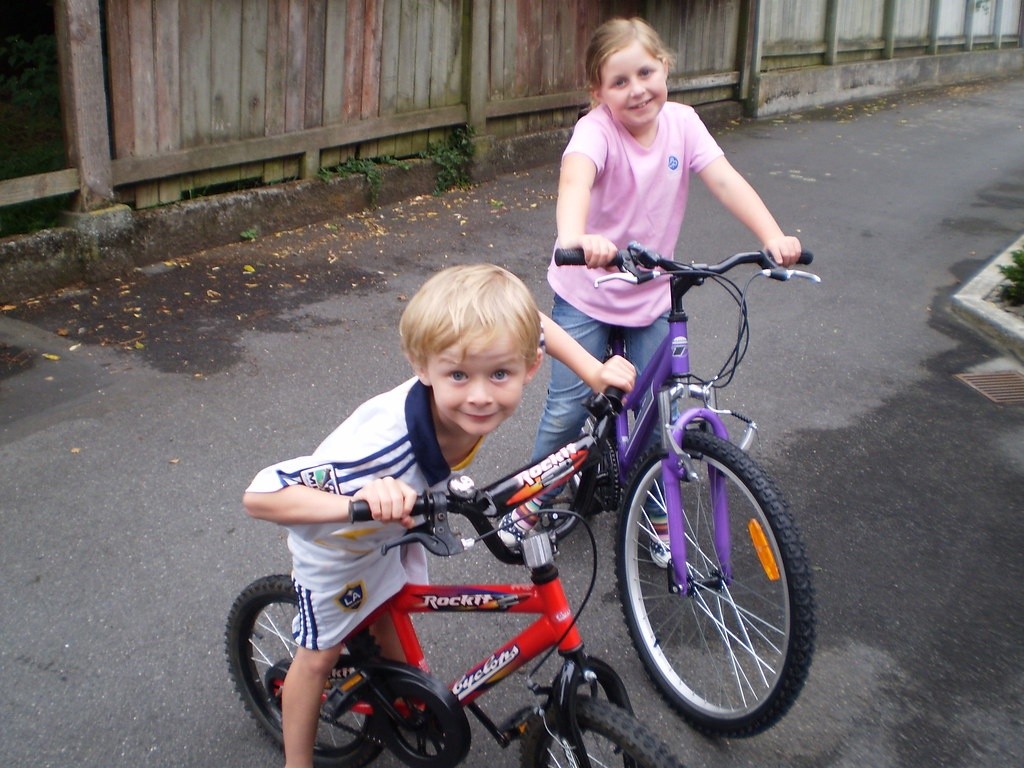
[
  {"x1": 496, "y1": 511, "x2": 527, "y2": 546},
  {"x1": 646, "y1": 517, "x2": 672, "y2": 569}
]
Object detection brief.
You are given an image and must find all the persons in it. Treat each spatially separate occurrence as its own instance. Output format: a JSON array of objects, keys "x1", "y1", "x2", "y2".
[
  {"x1": 242, "y1": 263, "x2": 638, "y2": 768},
  {"x1": 495, "y1": 14, "x2": 803, "y2": 570}
]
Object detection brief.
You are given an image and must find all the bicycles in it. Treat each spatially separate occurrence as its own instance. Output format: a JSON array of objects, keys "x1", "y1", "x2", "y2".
[
  {"x1": 553, "y1": 249, "x2": 820, "y2": 742},
  {"x1": 225, "y1": 384, "x2": 686, "y2": 768}
]
